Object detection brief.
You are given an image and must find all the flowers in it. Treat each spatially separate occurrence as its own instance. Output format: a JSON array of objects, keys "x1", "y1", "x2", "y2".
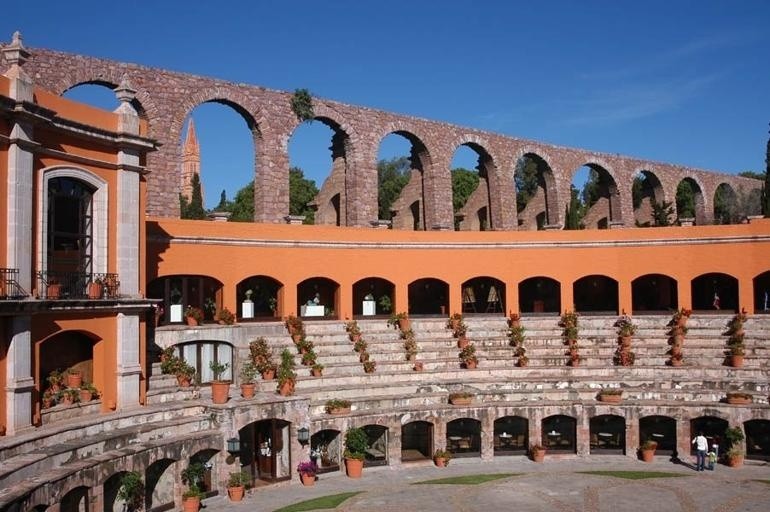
[
  {"x1": 637, "y1": 437, "x2": 656, "y2": 451},
  {"x1": 298, "y1": 462, "x2": 318, "y2": 476},
  {"x1": 46, "y1": 276, "x2": 64, "y2": 284},
  {"x1": 530, "y1": 441, "x2": 549, "y2": 456},
  {"x1": 101, "y1": 272, "x2": 122, "y2": 287}
]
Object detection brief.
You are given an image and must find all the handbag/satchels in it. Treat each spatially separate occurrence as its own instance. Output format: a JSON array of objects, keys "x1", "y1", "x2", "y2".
[{"x1": 694, "y1": 442, "x2": 697, "y2": 450}]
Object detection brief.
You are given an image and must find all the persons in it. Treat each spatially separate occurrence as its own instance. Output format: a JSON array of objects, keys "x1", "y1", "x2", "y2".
[
  {"x1": 707, "y1": 448, "x2": 717, "y2": 471},
  {"x1": 711, "y1": 432, "x2": 720, "y2": 457},
  {"x1": 713, "y1": 291, "x2": 721, "y2": 310},
  {"x1": 692, "y1": 430, "x2": 708, "y2": 471},
  {"x1": 313, "y1": 292, "x2": 320, "y2": 306}
]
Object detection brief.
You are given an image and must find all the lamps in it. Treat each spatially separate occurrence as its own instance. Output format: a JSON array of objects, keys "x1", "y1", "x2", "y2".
[
  {"x1": 297, "y1": 425, "x2": 310, "y2": 448},
  {"x1": 227, "y1": 435, "x2": 242, "y2": 458}
]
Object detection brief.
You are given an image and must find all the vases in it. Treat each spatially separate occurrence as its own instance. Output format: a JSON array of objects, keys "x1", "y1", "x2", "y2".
[
  {"x1": 107, "y1": 286, "x2": 118, "y2": 297},
  {"x1": 48, "y1": 285, "x2": 64, "y2": 299},
  {"x1": 301, "y1": 474, "x2": 316, "y2": 486},
  {"x1": 642, "y1": 450, "x2": 655, "y2": 461},
  {"x1": 534, "y1": 449, "x2": 546, "y2": 462}
]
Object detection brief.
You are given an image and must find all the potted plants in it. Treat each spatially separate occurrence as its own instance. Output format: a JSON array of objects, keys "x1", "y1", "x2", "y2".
[
  {"x1": 669, "y1": 306, "x2": 693, "y2": 366},
  {"x1": 727, "y1": 307, "x2": 751, "y2": 368},
  {"x1": 389, "y1": 314, "x2": 422, "y2": 371},
  {"x1": 614, "y1": 310, "x2": 636, "y2": 367},
  {"x1": 728, "y1": 391, "x2": 754, "y2": 404},
  {"x1": 449, "y1": 313, "x2": 479, "y2": 370},
  {"x1": 344, "y1": 427, "x2": 369, "y2": 478},
  {"x1": 507, "y1": 314, "x2": 529, "y2": 367},
  {"x1": 726, "y1": 425, "x2": 747, "y2": 468},
  {"x1": 448, "y1": 392, "x2": 475, "y2": 404},
  {"x1": 88, "y1": 279, "x2": 103, "y2": 299},
  {"x1": 327, "y1": 398, "x2": 354, "y2": 413},
  {"x1": 179, "y1": 463, "x2": 213, "y2": 511},
  {"x1": 154, "y1": 297, "x2": 296, "y2": 399},
  {"x1": 434, "y1": 447, "x2": 451, "y2": 468},
  {"x1": 562, "y1": 311, "x2": 583, "y2": 367},
  {"x1": 599, "y1": 390, "x2": 623, "y2": 401},
  {"x1": 226, "y1": 471, "x2": 257, "y2": 501},
  {"x1": 40, "y1": 364, "x2": 104, "y2": 408},
  {"x1": 345, "y1": 317, "x2": 375, "y2": 373},
  {"x1": 286, "y1": 315, "x2": 324, "y2": 376}
]
